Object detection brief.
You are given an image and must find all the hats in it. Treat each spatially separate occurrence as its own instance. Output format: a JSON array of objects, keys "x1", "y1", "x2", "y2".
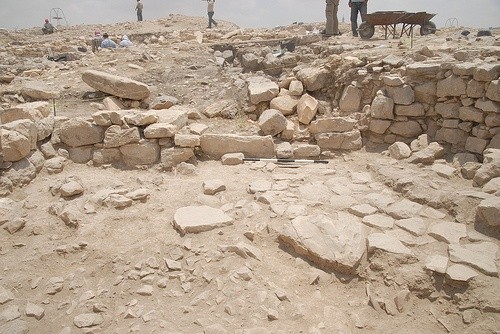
[
  {"x1": 95, "y1": 31, "x2": 100, "y2": 36},
  {"x1": 45, "y1": 19, "x2": 49, "y2": 22},
  {"x1": 103, "y1": 32, "x2": 108, "y2": 35}
]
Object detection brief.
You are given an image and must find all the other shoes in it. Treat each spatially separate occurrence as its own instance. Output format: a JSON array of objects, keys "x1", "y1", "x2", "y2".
[{"x1": 353, "y1": 33, "x2": 358, "y2": 37}]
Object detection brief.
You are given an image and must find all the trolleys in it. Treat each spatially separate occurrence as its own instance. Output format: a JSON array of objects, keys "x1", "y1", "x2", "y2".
[{"x1": 356, "y1": 11, "x2": 440, "y2": 40}]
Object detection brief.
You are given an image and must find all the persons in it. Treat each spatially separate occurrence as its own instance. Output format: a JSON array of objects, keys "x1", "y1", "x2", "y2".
[
  {"x1": 101, "y1": 33, "x2": 116, "y2": 48},
  {"x1": 348, "y1": 0, "x2": 368, "y2": 37},
  {"x1": 323, "y1": 0, "x2": 340, "y2": 36},
  {"x1": 206, "y1": 0, "x2": 217, "y2": 28},
  {"x1": 41, "y1": 18, "x2": 58, "y2": 34},
  {"x1": 135, "y1": 0, "x2": 143, "y2": 21},
  {"x1": 120, "y1": 35, "x2": 133, "y2": 47},
  {"x1": 92, "y1": 30, "x2": 104, "y2": 52}
]
